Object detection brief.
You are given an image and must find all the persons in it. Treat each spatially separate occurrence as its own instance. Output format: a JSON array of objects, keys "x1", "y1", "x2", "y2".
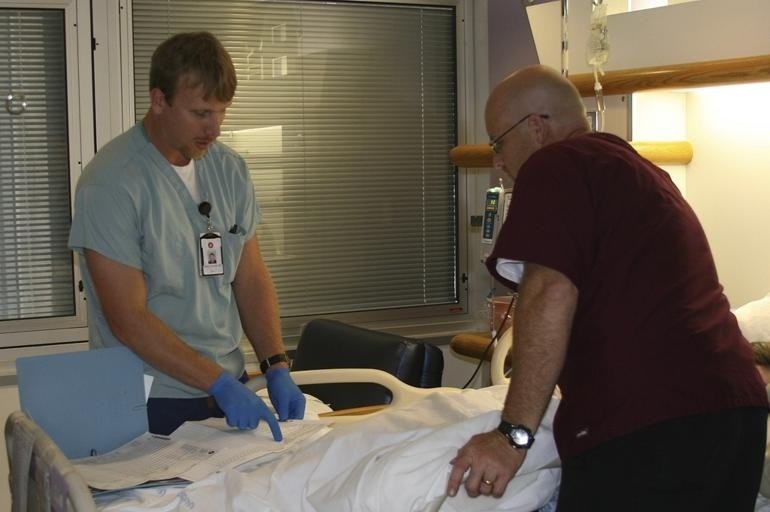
[
  {"x1": 446, "y1": 66, "x2": 770, "y2": 511},
  {"x1": 208, "y1": 253, "x2": 216, "y2": 264},
  {"x1": 68, "y1": 31, "x2": 306, "y2": 441}
]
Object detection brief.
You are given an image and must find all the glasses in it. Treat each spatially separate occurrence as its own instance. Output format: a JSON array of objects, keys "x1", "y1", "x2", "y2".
[{"x1": 489, "y1": 113, "x2": 549, "y2": 154}]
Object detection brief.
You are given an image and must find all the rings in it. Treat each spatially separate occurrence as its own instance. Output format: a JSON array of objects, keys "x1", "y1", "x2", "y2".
[{"x1": 482, "y1": 479, "x2": 492, "y2": 485}]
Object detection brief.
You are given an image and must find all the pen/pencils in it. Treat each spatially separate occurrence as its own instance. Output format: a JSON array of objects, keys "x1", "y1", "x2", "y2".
[{"x1": 229, "y1": 224, "x2": 238, "y2": 234}]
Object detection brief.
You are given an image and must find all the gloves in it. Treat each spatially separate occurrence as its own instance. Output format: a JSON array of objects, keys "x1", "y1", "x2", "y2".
[
  {"x1": 266, "y1": 368, "x2": 306, "y2": 422},
  {"x1": 206, "y1": 368, "x2": 282, "y2": 442}
]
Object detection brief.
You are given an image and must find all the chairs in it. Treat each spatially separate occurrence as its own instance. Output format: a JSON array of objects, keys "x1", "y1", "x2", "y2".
[{"x1": 286, "y1": 321, "x2": 442, "y2": 411}]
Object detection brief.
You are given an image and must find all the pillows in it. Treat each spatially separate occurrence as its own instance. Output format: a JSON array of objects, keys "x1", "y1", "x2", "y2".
[{"x1": 728, "y1": 291, "x2": 770, "y2": 343}]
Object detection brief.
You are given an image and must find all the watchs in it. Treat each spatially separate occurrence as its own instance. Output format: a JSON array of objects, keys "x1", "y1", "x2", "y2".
[{"x1": 497, "y1": 421, "x2": 535, "y2": 451}]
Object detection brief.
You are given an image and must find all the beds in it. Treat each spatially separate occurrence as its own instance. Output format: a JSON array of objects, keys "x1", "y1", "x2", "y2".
[{"x1": 3, "y1": 287, "x2": 770, "y2": 511}]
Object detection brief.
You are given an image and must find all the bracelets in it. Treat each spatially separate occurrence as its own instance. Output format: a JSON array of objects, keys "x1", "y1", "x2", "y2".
[{"x1": 260, "y1": 354, "x2": 292, "y2": 372}]
